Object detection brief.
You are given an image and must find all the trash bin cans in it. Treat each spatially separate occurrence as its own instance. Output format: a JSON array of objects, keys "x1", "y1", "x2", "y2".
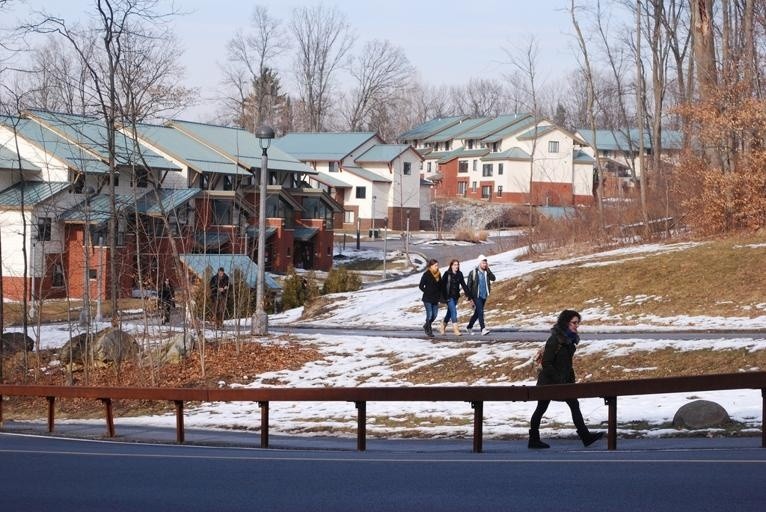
[{"x1": 369, "y1": 229, "x2": 380, "y2": 238}]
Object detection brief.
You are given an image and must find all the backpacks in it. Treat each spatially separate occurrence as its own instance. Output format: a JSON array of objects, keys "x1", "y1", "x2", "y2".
[{"x1": 533, "y1": 346, "x2": 545, "y2": 376}]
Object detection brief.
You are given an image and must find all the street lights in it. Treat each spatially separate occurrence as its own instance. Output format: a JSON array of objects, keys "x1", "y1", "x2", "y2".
[
  {"x1": 74, "y1": 184, "x2": 98, "y2": 330},
  {"x1": 405, "y1": 209, "x2": 412, "y2": 267},
  {"x1": 381, "y1": 216, "x2": 390, "y2": 283},
  {"x1": 91, "y1": 221, "x2": 108, "y2": 322},
  {"x1": 371, "y1": 195, "x2": 376, "y2": 241},
  {"x1": 248, "y1": 124, "x2": 275, "y2": 337}
]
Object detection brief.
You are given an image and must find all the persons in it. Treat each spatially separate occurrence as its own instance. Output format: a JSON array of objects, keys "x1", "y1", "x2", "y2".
[
  {"x1": 208, "y1": 266, "x2": 231, "y2": 329},
  {"x1": 527, "y1": 308, "x2": 605, "y2": 450},
  {"x1": 418, "y1": 258, "x2": 450, "y2": 338},
  {"x1": 463, "y1": 254, "x2": 497, "y2": 337},
  {"x1": 156, "y1": 276, "x2": 176, "y2": 325},
  {"x1": 438, "y1": 258, "x2": 474, "y2": 336}
]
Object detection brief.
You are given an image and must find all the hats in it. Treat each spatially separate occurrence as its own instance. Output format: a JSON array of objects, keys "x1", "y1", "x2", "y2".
[{"x1": 479, "y1": 255, "x2": 487, "y2": 261}]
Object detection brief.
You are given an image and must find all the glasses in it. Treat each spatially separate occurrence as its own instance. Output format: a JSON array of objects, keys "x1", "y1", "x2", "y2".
[{"x1": 571, "y1": 321, "x2": 578, "y2": 325}]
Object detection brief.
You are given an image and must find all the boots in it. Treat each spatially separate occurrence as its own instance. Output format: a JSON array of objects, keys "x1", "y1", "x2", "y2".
[
  {"x1": 438, "y1": 321, "x2": 463, "y2": 336},
  {"x1": 528, "y1": 429, "x2": 550, "y2": 448},
  {"x1": 577, "y1": 425, "x2": 603, "y2": 446},
  {"x1": 424, "y1": 319, "x2": 435, "y2": 338}
]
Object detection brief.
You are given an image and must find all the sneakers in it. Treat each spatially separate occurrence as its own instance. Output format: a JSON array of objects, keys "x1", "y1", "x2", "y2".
[{"x1": 465, "y1": 327, "x2": 490, "y2": 336}]
